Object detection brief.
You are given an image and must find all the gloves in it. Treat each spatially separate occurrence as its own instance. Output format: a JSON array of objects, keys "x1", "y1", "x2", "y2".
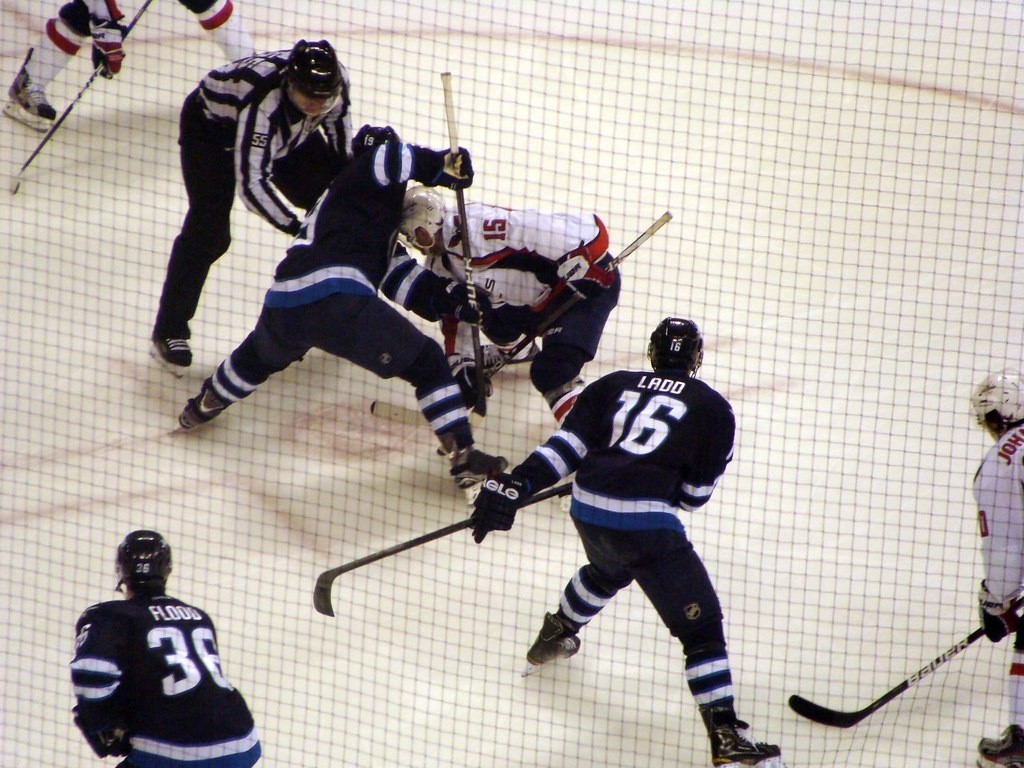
[
  {"x1": 428, "y1": 276, "x2": 492, "y2": 323},
  {"x1": 568, "y1": 264, "x2": 613, "y2": 301},
  {"x1": 453, "y1": 365, "x2": 483, "y2": 405},
  {"x1": 469, "y1": 474, "x2": 525, "y2": 543},
  {"x1": 411, "y1": 146, "x2": 473, "y2": 189},
  {"x1": 88, "y1": 19, "x2": 125, "y2": 79},
  {"x1": 974, "y1": 580, "x2": 1022, "y2": 642}
]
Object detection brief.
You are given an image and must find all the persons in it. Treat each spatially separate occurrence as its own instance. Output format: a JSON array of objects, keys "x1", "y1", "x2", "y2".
[
  {"x1": 8, "y1": 0, "x2": 260, "y2": 121},
  {"x1": 149, "y1": 37, "x2": 357, "y2": 368},
  {"x1": 177, "y1": 123, "x2": 510, "y2": 491},
  {"x1": 467, "y1": 316, "x2": 782, "y2": 768},
  {"x1": 395, "y1": 185, "x2": 622, "y2": 428},
  {"x1": 970, "y1": 366, "x2": 1024, "y2": 768},
  {"x1": 68, "y1": 529, "x2": 262, "y2": 768}
]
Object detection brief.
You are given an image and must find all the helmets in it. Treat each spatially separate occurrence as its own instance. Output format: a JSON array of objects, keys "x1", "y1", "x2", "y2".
[
  {"x1": 349, "y1": 123, "x2": 401, "y2": 156},
  {"x1": 114, "y1": 530, "x2": 174, "y2": 586},
  {"x1": 398, "y1": 184, "x2": 446, "y2": 244},
  {"x1": 970, "y1": 367, "x2": 1023, "y2": 438},
  {"x1": 285, "y1": 39, "x2": 342, "y2": 117},
  {"x1": 649, "y1": 318, "x2": 704, "y2": 377}
]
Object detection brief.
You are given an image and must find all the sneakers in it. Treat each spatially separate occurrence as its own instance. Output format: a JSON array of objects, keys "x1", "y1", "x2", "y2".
[
  {"x1": 174, "y1": 379, "x2": 227, "y2": 431},
  {"x1": 976, "y1": 725, "x2": 1023, "y2": 768},
  {"x1": 446, "y1": 438, "x2": 509, "y2": 505},
  {"x1": 702, "y1": 711, "x2": 787, "y2": 768},
  {"x1": 2, "y1": 47, "x2": 56, "y2": 134},
  {"x1": 520, "y1": 611, "x2": 581, "y2": 678},
  {"x1": 149, "y1": 333, "x2": 192, "y2": 377}
]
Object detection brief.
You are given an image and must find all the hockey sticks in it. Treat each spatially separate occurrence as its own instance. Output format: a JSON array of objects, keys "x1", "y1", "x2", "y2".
[
  {"x1": 9, "y1": 0, "x2": 151, "y2": 196},
  {"x1": 440, "y1": 69, "x2": 490, "y2": 428},
  {"x1": 313, "y1": 482, "x2": 574, "y2": 617},
  {"x1": 789, "y1": 597, "x2": 1024, "y2": 731},
  {"x1": 369, "y1": 209, "x2": 673, "y2": 430}
]
